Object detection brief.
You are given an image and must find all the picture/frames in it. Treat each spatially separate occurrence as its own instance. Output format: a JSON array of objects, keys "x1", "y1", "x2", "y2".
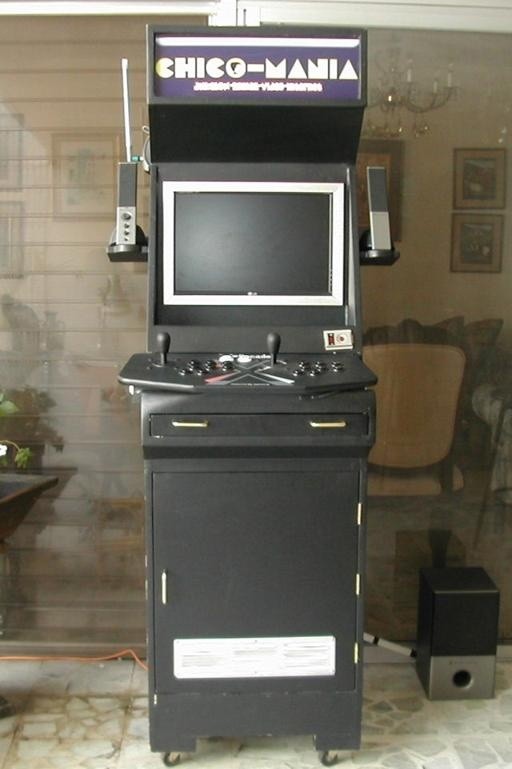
[
  {"x1": 355, "y1": 137, "x2": 404, "y2": 243},
  {"x1": 0, "y1": 109, "x2": 123, "y2": 279},
  {"x1": 449, "y1": 145, "x2": 506, "y2": 276}
]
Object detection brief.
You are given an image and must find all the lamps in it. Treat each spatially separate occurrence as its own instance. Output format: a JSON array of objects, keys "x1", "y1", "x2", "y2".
[{"x1": 361, "y1": 29, "x2": 459, "y2": 143}]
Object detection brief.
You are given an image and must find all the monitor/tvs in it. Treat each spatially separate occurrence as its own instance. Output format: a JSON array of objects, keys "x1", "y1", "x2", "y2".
[{"x1": 161, "y1": 180, "x2": 345, "y2": 308}]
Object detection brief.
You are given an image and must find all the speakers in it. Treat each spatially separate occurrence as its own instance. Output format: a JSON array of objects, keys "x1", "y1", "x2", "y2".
[
  {"x1": 414, "y1": 565, "x2": 501, "y2": 699},
  {"x1": 367, "y1": 166, "x2": 392, "y2": 250},
  {"x1": 115, "y1": 162, "x2": 138, "y2": 245}
]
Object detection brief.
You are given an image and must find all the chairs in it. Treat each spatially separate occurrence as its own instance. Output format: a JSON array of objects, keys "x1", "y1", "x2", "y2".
[{"x1": 354, "y1": 340, "x2": 466, "y2": 564}]
